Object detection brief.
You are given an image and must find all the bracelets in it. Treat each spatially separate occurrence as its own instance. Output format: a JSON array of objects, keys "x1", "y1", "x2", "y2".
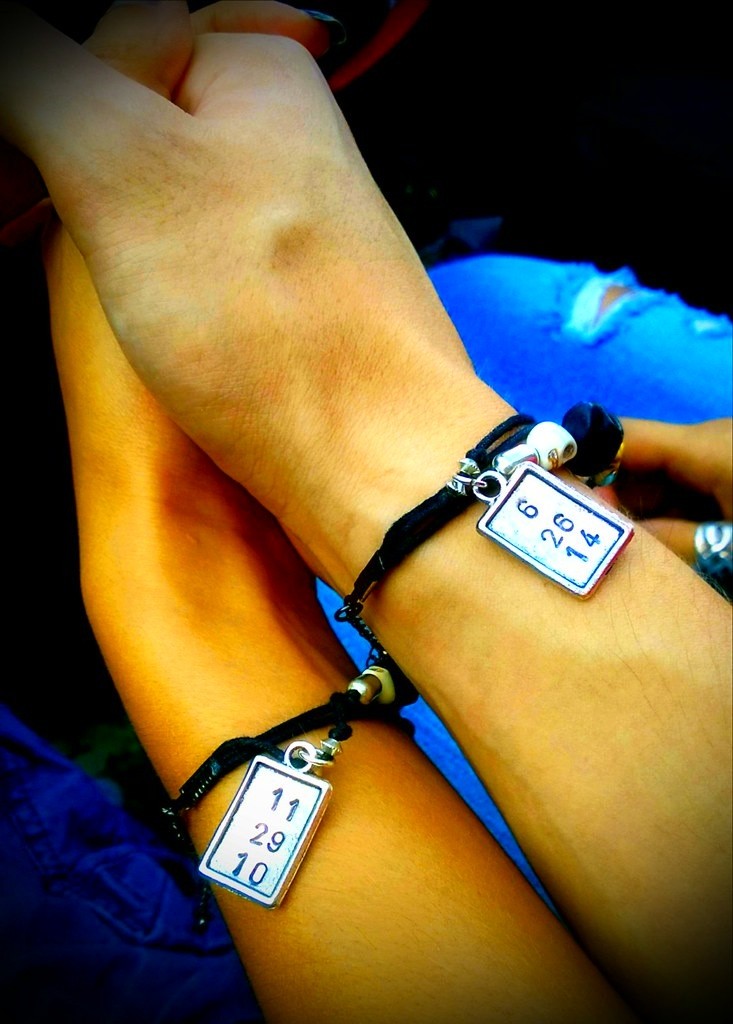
[
  {"x1": 334, "y1": 396, "x2": 628, "y2": 633},
  {"x1": 132, "y1": 652, "x2": 416, "y2": 925}
]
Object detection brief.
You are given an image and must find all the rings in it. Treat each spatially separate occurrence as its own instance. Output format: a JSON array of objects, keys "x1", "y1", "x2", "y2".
[{"x1": 679, "y1": 514, "x2": 731, "y2": 600}]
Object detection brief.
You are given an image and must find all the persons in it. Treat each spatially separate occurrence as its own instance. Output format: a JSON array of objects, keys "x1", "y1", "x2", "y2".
[
  {"x1": 0, "y1": 0, "x2": 732, "y2": 1024},
  {"x1": 49, "y1": 0, "x2": 732, "y2": 1024}
]
